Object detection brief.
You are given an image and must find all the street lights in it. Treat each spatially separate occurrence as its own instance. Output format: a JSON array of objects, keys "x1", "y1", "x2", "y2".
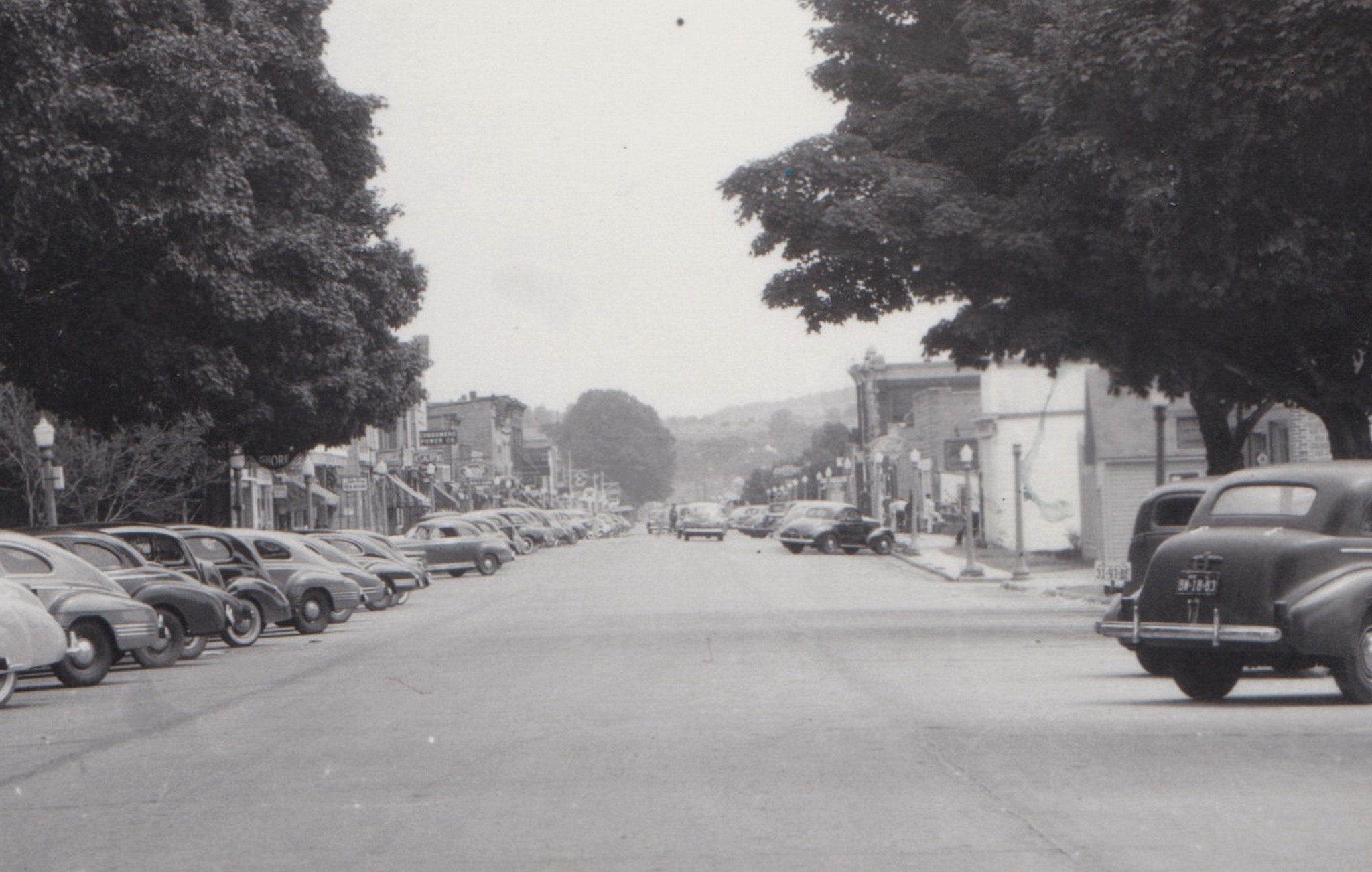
[
  {"x1": 425, "y1": 463, "x2": 436, "y2": 513},
  {"x1": 32, "y1": 416, "x2": 58, "y2": 526},
  {"x1": 376, "y1": 460, "x2": 390, "y2": 534},
  {"x1": 229, "y1": 449, "x2": 245, "y2": 527}
]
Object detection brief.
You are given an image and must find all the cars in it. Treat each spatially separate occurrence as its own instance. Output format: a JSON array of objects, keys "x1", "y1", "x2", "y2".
[
  {"x1": 387, "y1": 506, "x2": 632, "y2": 575},
  {"x1": 1093, "y1": 459, "x2": 1371, "y2": 707},
  {"x1": 0, "y1": 520, "x2": 433, "y2": 707},
  {"x1": 676, "y1": 499, "x2": 897, "y2": 556}
]
